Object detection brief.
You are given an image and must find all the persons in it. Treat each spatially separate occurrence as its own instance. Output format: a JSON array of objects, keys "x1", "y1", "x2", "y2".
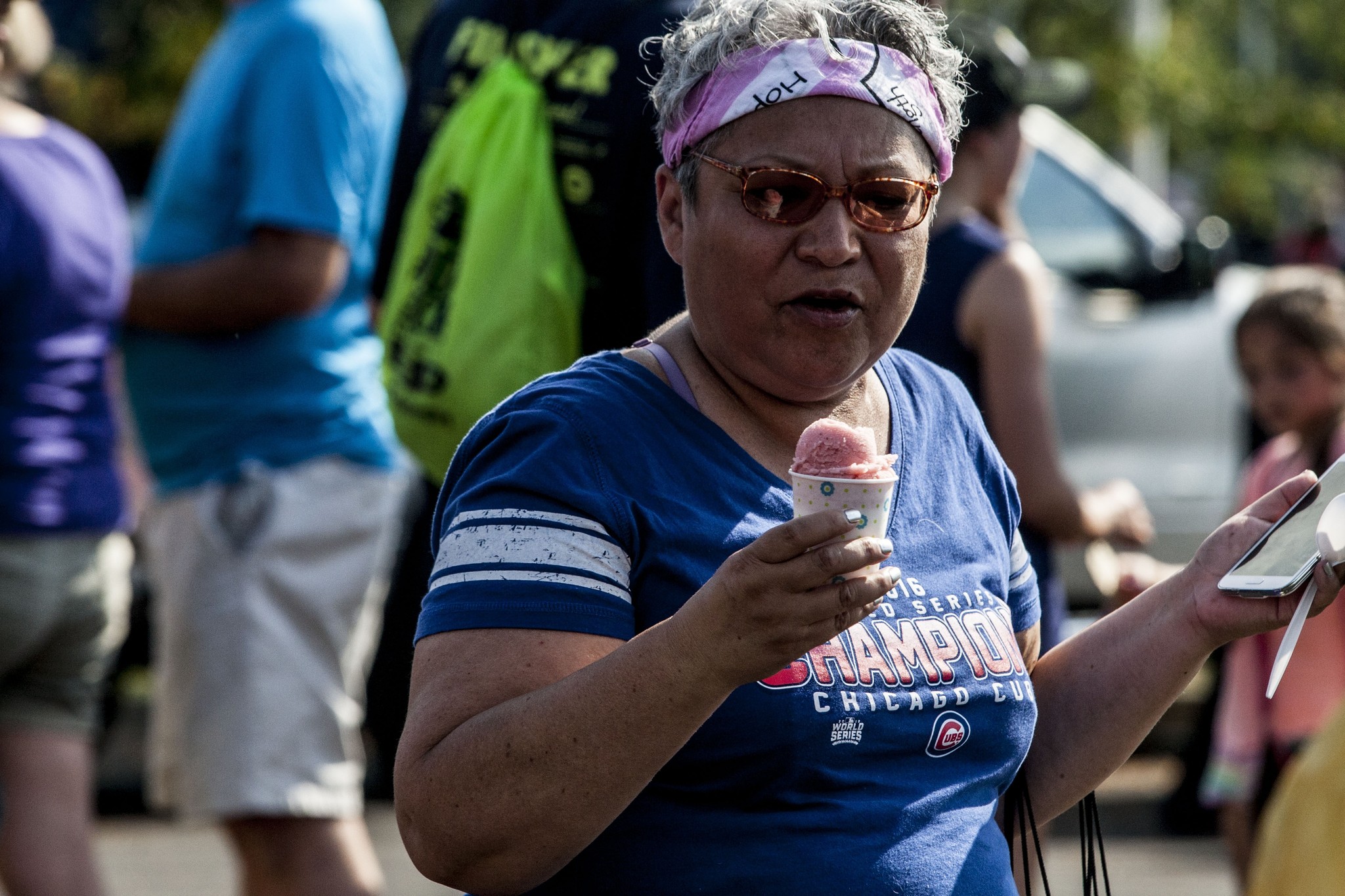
[
  {"x1": 394, "y1": 1, "x2": 1345, "y2": 896},
  {"x1": 1, "y1": 0, "x2": 1345, "y2": 896}
]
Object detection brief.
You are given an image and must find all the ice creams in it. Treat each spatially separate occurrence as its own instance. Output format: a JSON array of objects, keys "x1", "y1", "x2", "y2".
[{"x1": 791, "y1": 417, "x2": 898, "y2": 482}]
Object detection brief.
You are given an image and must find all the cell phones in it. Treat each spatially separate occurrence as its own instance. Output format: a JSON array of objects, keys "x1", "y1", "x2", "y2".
[{"x1": 1216, "y1": 452, "x2": 1345, "y2": 599}]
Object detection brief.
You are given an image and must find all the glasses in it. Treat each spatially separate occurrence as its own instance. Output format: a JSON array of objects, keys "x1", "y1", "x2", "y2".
[{"x1": 689, "y1": 146, "x2": 940, "y2": 232}]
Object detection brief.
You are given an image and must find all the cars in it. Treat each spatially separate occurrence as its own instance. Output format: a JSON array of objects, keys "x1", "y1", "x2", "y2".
[{"x1": 1001, "y1": 103, "x2": 1345, "y2": 572}]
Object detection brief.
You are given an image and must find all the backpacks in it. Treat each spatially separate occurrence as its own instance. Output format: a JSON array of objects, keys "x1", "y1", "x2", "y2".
[{"x1": 376, "y1": 0, "x2": 644, "y2": 483}]
